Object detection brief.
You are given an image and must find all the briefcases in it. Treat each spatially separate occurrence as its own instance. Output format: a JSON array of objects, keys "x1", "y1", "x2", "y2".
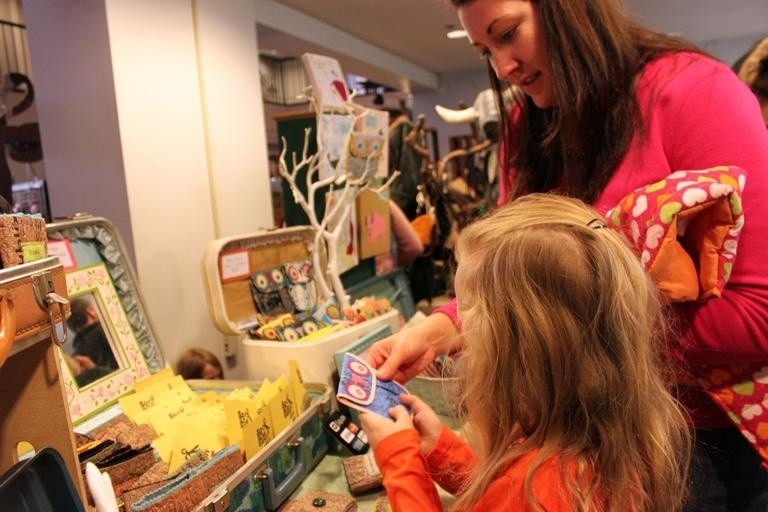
[{"x1": 0, "y1": 256, "x2": 74, "y2": 370}]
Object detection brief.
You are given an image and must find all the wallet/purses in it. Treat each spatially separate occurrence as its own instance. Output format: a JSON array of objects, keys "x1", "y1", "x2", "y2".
[
  {"x1": 335, "y1": 350, "x2": 411, "y2": 424},
  {"x1": 277, "y1": 449, "x2": 395, "y2": 512}
]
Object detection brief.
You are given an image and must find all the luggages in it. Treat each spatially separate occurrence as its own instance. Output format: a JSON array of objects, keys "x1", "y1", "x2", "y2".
[{"x1": 38, "y1": 212, "x2": 334, "y2": 511}]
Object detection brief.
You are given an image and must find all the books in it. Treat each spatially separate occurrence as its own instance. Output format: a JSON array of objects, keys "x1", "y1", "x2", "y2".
[{"x1": 299, "y1": 51, "x2": 392, "y2": 275}]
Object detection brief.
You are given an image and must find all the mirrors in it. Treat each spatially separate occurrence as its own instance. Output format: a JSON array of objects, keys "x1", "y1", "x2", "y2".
[{"x1": 56, "y1": 263, "x2": 150, "y2": 427}]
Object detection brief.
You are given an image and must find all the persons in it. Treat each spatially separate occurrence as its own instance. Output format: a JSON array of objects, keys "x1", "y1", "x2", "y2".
[
  {"x1": 174, "y1": 346, "x2": 224, "y2": 381},
  {"x1": 356, "y1": 189, "x2": 692, "y2": 512},
  {"x1": 344, "y1": 0, "x2": 768, "y2": 512},
  {"x1": 61, "y1": 298, "x2": 119, "y2": 370},
  {"x1": 59, "y1": 350, "x2": 115, "y2": 390}
]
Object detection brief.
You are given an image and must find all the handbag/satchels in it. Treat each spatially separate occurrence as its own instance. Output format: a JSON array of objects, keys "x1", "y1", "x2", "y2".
[{"x1": 71, "y1": 418, "x2": 246, "y2": 511}]
